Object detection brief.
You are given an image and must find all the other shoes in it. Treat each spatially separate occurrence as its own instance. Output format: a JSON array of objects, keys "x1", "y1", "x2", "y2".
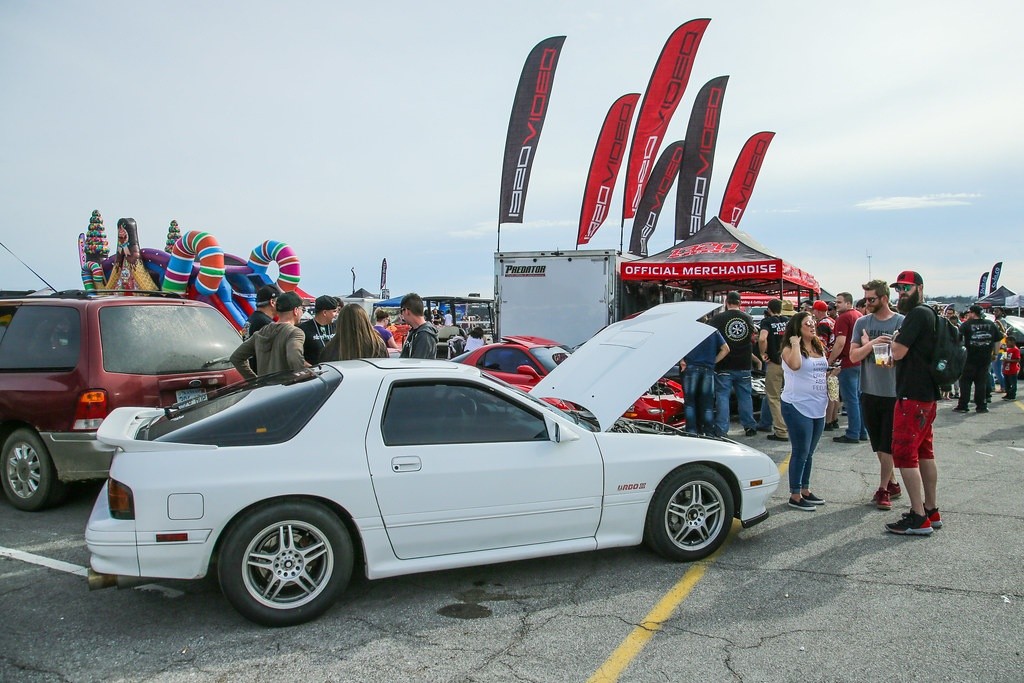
[
  {"x1": 977, "y1": 408, "x2": 989, "y2": 413},
  {"x1": 746, "y1": 428, "x2": 756, "y2": 436},
  {"x1": 995, "y1": 388, "x2": 1006, "y2": 392},
  {"x1": 1002, "y1": 395, "x2": 1017, "y2": 401},
  {"x1": 833, "y1": 435, "x2": 867, "y2": 443},
  {"x1": 756, "y1": 427, "x2": 771, "y2": 432},
  {"x1": 824, "y1": 419, "x2": 839, "y2": 431},
  {"x1": 953, "y1": 407, "x2": 967, "y2": 413},
  {"x1": 842, "y1": 406, "x2": 848, "y2": 416},
  {"x1": 970, "y1": 397, "x2": 991, "y2": 403},
  {"x1": 767, "y1": 433, "x2": 788, "y2": 441}
]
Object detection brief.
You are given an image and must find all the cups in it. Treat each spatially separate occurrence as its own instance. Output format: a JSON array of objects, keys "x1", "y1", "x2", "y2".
[
  {"x1": 872, "y1": 343, "x2": 888, "y2": 365},
  {"x1": 1002, "y1": 352, "x2": 1007, "y2": 359}
]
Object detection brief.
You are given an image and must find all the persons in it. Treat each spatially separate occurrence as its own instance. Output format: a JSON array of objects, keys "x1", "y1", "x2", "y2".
[
  {"x1": 884, "y1": 269, "x2": 943, "y2": 534},
  {"x1": 849, "y1": 278, "x2": 908, "y2": 510},
  {"x1": 230, "y1": 284, "x2": 486, "y2": 381},
  {"x1": 678, "y1": 291, "x2": 1021, "y2": 443},
  {"x1": 780, "y1": 312, "x2": 829, "y2": 511}
]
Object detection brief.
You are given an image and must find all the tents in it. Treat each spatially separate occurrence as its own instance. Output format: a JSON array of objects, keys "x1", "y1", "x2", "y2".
[
  {"x1": 973, "y1": 285, "x2": 1024, "y2": 317},
  {"x1": 618, "y1": 216, "x2": 820, "y2": 324}
]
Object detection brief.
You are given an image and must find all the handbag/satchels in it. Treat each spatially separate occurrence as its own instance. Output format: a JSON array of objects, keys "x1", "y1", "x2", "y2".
[{"x1": 827, "y1": 374, "x2": 840, "y2": 402}]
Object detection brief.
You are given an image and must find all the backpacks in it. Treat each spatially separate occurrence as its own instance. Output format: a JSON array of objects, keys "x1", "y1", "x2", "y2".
[{"x1": 915, "y1": 302, "x2": 967, "y2": 392}]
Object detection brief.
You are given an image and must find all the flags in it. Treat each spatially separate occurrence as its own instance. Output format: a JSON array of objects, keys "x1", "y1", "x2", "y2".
[
  {"x1": 672, "y1": 75, "x2": 730, "y2": 241},
  {"x1": 989, "y1": 262, "x2": 1003, "y2": 294},
  {"x1": 575, "y1": 94, "x2": 640, "y2": 246},
  {"x1": 717, "y1": 131, "x2": 775, "y2": 232},
  {"x1": 623, "y1": 17, "x2": 711, "y2": 218},
  {"x1": 978, "y1": 272, "x2": 990, "y2": 297},
  {"x1": 628, "y1": 139, "x2": 687, "y2": 258},
  {"x1": 499, "y1": 34, "x2": 567, "y2": 223}
]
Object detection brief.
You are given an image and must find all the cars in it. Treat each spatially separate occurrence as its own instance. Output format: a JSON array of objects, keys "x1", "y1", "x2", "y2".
[
  {"x1": 84, "y1": 301, "x2": 780, "y2": 629},
  {"x1": 451, "y1": 335, "x2": 686, "y2": 433},
  {"x1": 663, "y1": 363, "x2": 766, "y2": 419},
  {"x1": 749, "y1": 306, "x2": 768, "y2": 324},
  {"x1": 983, "y1": 313, "x2": 1024, "y2": 380}
]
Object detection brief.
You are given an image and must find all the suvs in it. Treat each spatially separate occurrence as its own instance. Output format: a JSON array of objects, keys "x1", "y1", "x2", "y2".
[{"x1": 0, "y1": 289, "x2": 245, "y2": 512}]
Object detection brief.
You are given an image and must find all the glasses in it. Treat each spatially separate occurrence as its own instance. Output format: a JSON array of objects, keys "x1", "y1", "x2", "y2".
[
  {"x1": 806, "y1": 320, "x2": 817, "y2": 326},
  {"x1": 863, "y1": 296, "x2": 878, "y2": 303},
  {"x1": 895, "y1": 284, "x2": 913, "y2": 292},
  {"x1": 401, "y1": 308, "x2": 410, "y2": 315}
]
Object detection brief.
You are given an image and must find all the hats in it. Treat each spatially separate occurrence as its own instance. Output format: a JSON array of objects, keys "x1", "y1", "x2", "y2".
[
  {"x1": 970, "y1": 306, "x2": 987, "y2": 319},
  {"x1": 810, "y1": 300, "x2": 828, "y2": 311},
  {"x1": 726, "y1": 292, "x2": 740, "y2": 303},
  {"x1": 889, "y1": 270, "x2": 923, "y2": 287},
  {"x1": 256, "y1": 284, "x2": 281, "y2": 303},
  {"x1": 781, "y1": 300, "x2": 799, "y2": 316}
]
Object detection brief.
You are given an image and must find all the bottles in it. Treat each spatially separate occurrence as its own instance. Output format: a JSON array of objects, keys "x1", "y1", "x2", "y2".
[{"x1": 936, "y1": 359, "x2": 947, "y2": 371}]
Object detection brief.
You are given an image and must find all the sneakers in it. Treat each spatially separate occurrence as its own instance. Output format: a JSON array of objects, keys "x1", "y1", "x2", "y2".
[
  {"x1": 873, "y1": 480, "x2": 902, "y2": 500},
  {"x1": 801, "y1": 493, "x2": 825, "y2": 504},
  {"x1": 885, "y1": 507, "x2": 934, "y2": 535},
  {"x1": 788, "y1": 497, "x2": 817, "y2": 511},
  {"x1": 875, "y1": 487, "x2": 892, "y2": 510},
  {"x1": 901, "y1": 503, "x2": 942, "y2": 528}
]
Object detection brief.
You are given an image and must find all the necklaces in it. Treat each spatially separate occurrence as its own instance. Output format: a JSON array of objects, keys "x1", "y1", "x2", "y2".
[{"x1": 313, "y1": 318, "x2": 331, "y2": 347}]
